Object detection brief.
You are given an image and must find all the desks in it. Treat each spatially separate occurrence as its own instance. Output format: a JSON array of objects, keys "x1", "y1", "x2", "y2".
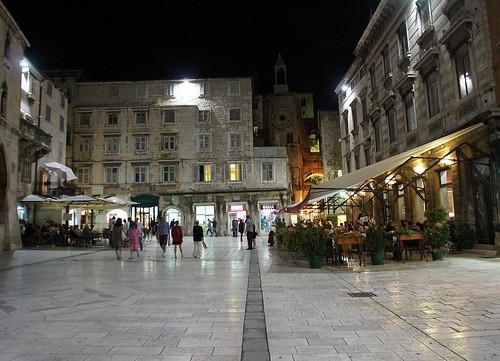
[
  {"x1": 332, "y1": 233, "x2": 365, "y2": 269},
  {"x1": 397, "y1": 233, "x2": 430, "y2": 262}
]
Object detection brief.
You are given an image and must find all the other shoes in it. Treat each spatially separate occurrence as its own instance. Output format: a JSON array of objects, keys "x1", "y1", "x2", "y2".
[
  {"x1": 253, "y1": 246, "x2": 256, "y2": 249},
  {"x1": 175, "y1": 257, "x2": 177, "y2": 258},
  {"x1": 137, "y1": 254, "x2": 139, "y2": 258},
  {"x1": 268, "y1": 244, "x2": 273, "y2": 246},
  {"x1": 194, "y1": 255, "x2": 196, "y2": 258},
  {"x1": 117, "y1": 256, "x2": 119, "y2": 260},
  {"x1": 162, "y1": 253, "x2": 165, "y2": 257},
  {"x1": 246, "y1": 249, "x2": 252, "y2": 250},
  {"x1": 129, "y1": 256, "x2": 134, "y2": 259},
  {"x1": 181, "y1": 254, "x2": 183, "y2": 257},
  {"x1": 118, "y1": 256, "x2": 123, "y2": 259}
]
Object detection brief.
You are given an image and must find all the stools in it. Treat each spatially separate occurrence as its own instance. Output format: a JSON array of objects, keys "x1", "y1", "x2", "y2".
[{"x1": 70, "y1": 238, "x2": 89, "y2": 248}]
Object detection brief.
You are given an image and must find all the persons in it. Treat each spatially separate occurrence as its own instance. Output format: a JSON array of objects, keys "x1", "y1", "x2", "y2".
[
  {"x1": 170, "y1": 219, "x2": 183, "y2": 258},
  {"x1": 245, "y1": 215, "x2": 256, "y2": 250},
  {"x1": 232, "y1": 220, "x2": 238, "y2": 237},
  {"x1": 281, "y1": 214, "x2": 465, "y2": 263},
  {"x1": 193, "y1": 221, "x2": 204, "y2": 258},
  {"x1": 20, "y1": 220, "x2": 96, "y2": 246},
  {"x1": 268, "y1": 231, "x2": 274, "y2": 246},
  {"x1": 150, "y1": 215, "x2": 170, "y2": 257},
  {"x1": 110, "y1": 216, "x2": 143, "y2": 259},
  {"x1": 206, "y1": 218, "x2": 218, "y2": 237},
  {"x1": 239, "y1": 219, "x2": 245, "y2": 241}
]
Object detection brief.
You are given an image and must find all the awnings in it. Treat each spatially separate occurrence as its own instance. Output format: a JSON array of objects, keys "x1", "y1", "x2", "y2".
[
  {"x1": 271, "y1": 122, "x2": 492, "y2": 213},
  {"x1": 18, "y1": 194, "x2": 140, "y2": 206}
]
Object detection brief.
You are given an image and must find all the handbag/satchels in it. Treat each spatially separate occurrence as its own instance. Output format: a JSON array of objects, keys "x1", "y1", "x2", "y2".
[
  {"x1": 168, "y1": 240, "x2": 171, "y2": 246},
  {"x1": 202, "y1": 238, "x2": 207, "y2": 249}
]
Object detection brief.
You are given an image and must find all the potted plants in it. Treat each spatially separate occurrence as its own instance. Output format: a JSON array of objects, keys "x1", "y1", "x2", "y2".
[
  {"x1": 426, "y1": 225, "x2": 450, "y2": 261},
  {"x1": 303, "y1": 228, "x2": 329, "y2": 268},
  {"x1": 366, "y1": 226, "x2": 391, "y2": 265}
]
